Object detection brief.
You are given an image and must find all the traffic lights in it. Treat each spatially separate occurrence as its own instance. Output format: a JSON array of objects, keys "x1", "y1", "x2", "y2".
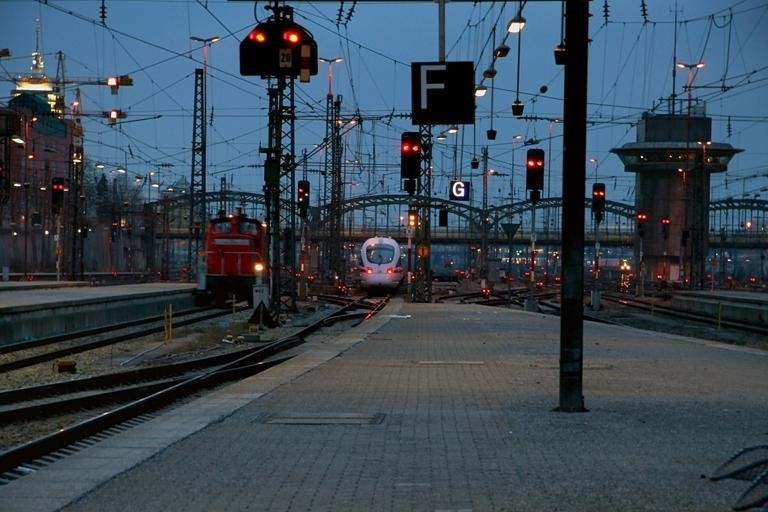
[
  {"x1": 111, "y1": 219, "x2": 118, "y2": 232},
  {"x1": 408, "y1": 210, "x2": 417, "y2": 228},
  {"x1": 592, "y1": 183, "x2": 605, "y2": 212},
  {"x1": 51, "y1": 177, "x2": 64, "y2": 208},
  {"x1": 526, "y1": 148, "x2": 544, "y2": 190},
  {"x1": 239, "y1": 20, "x2": 319, "y2": 78},
  {"x1": 660, "y1": 217, "x2": 670, "y2": 240},
  {"x1": 400, "y1": 131, "x2": 420, "y2": 180},
  {"x1": 297, "y1": 180, "x2": 310, "y2": 209}
]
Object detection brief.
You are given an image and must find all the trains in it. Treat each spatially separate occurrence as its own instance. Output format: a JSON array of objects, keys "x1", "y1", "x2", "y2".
[
  {"x1": 204, "y1": 207, "x2": 266, "y2": 309},
  {"x1": 360, "y1": 238, "x2": 405, "y2": 297}
]
